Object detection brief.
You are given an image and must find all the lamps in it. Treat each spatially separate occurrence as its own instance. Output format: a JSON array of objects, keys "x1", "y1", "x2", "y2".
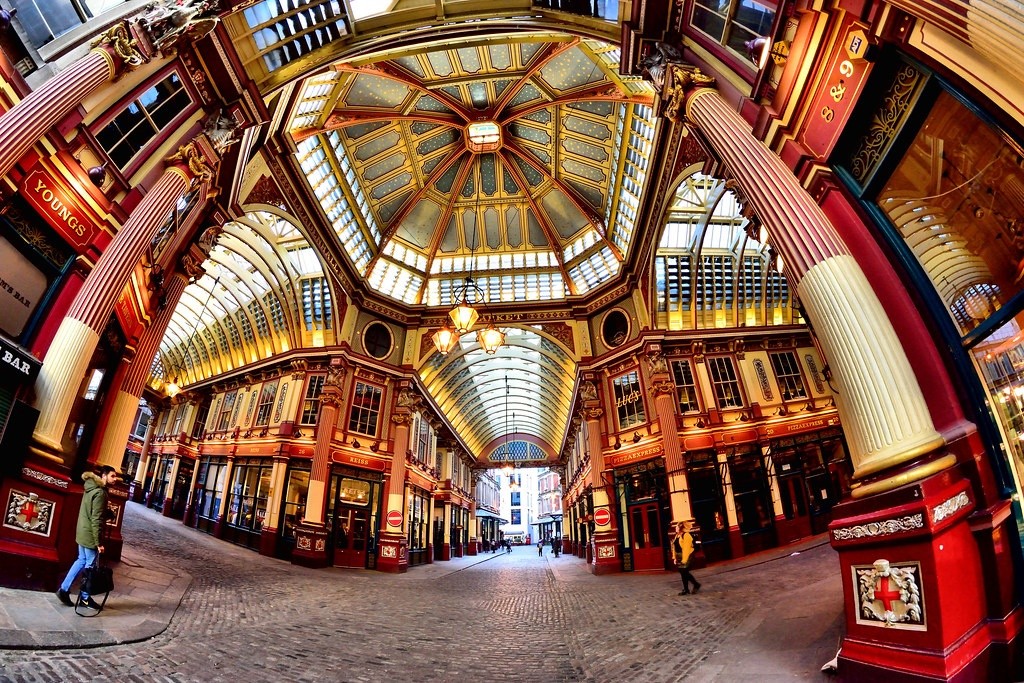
[
  {"x1": 613, "y1": 433, "x2": 625, "y2": 451},
  {"x1": 509, "y1": 412, "x2": 519, "y2": 491},
  {"x1": 800, "y1": 403, "x2": 813, "y2": 411},
  {"x1": 293, "y1": 425, "x2": 304, "y2": 438},
  {"x1": 351, "y1": 437, "x2": 360, "y2": 448},
  {"x1": 370, "y1": 439, "x2": 382, "y2": 452},
  {"x1": 633, "y1": 431, "x2": 643, "y2": 444},
  {"x1": 455, "y1": 486, "x2": 477, "y2": 502},
  {"x1": 149, "y1": 428, "x2": 270, "y2": 443},
  {"x1": 693, "y1": 417, "x2": 704, "y2": 427},
  {"x1": 744, "y1": 37, "x2": 788, "y2": 72},
  {"x1": 735, "y1": 412, "x2": 749, "y2": 422},
  {"x1": 413, "y1": 457, "x2": 441, "y2": 481},
  {"x1": 773, "y1": 406, "x2": 786, "y2": 416},
  {"x1": 825, "y1": 397, "x2": 836, "y2": 406},
  {"x1": 499, "y1": 376, "x2": 515, "y2": 477},
  {"x1": 431, "y1": 134, "x2": 507, "y2": 355},
  {"x1": 936, "y1": 149, "x2": 1017, "y2": 235},
  {"x1": 87, "y1": 161, "x2": 220, "y2": 399}
]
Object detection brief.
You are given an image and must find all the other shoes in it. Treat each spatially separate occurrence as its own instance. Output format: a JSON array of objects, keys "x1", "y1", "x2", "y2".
[
  {"x1": 679, "y1": 590, "x2": 690, "y2": 595},
  {"x1": 79, "y1": 596, "x2": 103, "y2": 610},
  {"x1": 56, "y1": 588, "x2": 74, "y2": 607},
  {"x1": 692, "y1": 583, "x2": 700, "y2": 594}
]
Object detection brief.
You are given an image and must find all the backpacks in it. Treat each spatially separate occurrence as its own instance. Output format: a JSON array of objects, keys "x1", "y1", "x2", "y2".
[{"x1": 682, "y1": 532, "x2": 701, "y2": 552}]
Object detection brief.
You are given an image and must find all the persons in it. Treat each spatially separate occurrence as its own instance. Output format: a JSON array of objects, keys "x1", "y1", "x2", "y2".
[
  {"x1": 672, "y1": 521, "x2": 701, "y2": 596},
  {"x1": 55, "y1": 465, "x2": 117, "y2": 611},
  {"x1": 537, "y1": 539, "x2": 544, "y2": 557},
  {"x1": 549, "y1": 536, "x2": 559, "y2": 558},
  {"x1": 485, "y1": 538, "x2": 513, "y2": 553}
]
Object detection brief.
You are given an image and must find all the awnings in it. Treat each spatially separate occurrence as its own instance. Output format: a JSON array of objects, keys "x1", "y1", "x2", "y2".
[
  {"x1": 530, "y1": 517, "x2": 556, "y2": 526},
  {"x1": 476, "y1": 510, "x2": 509, "y2": 526}
]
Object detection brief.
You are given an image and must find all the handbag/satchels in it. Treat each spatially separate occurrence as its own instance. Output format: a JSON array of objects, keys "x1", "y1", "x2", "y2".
[{"x1": 75, "y1": 552, "x2": 114, "y2": 616}]
